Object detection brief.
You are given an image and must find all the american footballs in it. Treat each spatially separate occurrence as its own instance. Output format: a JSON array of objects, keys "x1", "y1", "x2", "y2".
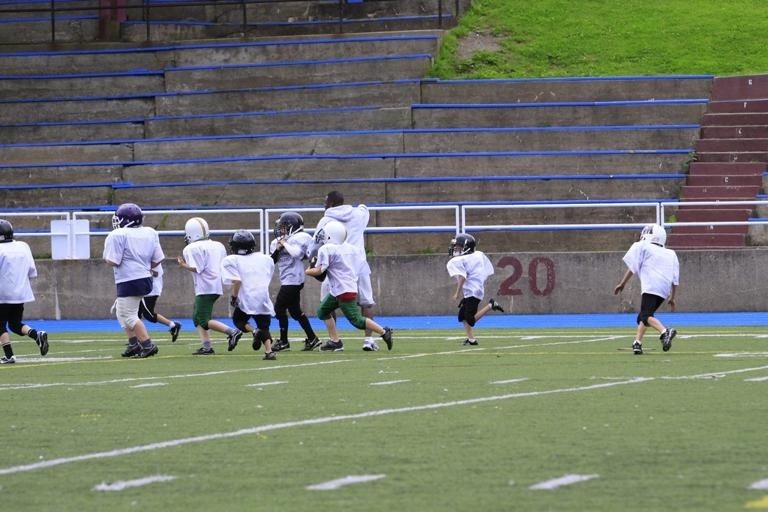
[{"x1": 309, "y1": 256, "x2": 327, "y2": 282}]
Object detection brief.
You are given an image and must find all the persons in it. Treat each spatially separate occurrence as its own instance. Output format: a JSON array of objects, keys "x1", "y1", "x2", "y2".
[
  {"x1": 613, "y1": 222, "x2": 680, "y2": 355},
  {"x1": 308, "y1": 191, "x2": 381, "y2": 352},
  {"x1": 0, "y1": 218, "x2": 50, "y2": 364},
  {"x1": 302, "y1": 221, "x2": 393, "y2": 353},
  {"x1": 175, "y1": 216, "x2": 244, "y2": 356},
  {"x1": 269, "y1": 211, "x2": 322, "y2": 352},
  {"x1": 137, "y1": 261, "x2": 183, "y2": 343},
  {"x1": 101, "y1": 203, "x2": 166, "y2": 359},
  {"x1": 445, "y1": 232, "x2": 505, "y2": 346},
  {"x1": 217, "y1": 229, "x2": 279, "y2": 361}
]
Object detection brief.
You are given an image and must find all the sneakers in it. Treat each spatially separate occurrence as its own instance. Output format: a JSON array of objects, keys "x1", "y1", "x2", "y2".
[
  {"x1": 252, "y1": 328, "x2": 264, "y2": 350},
  {"x1": 228, "y1": 329, "x2": 243, "y2": 351},
  {"x1": 659, "y1": 327, "x2": 677, "y2": 352},
  {"x1": 632, "y1": 341, "x2": 642, "y2": 353},
  {"x1": 170, "y1": 322, "x2": 181, "y2": 341},
  {"x1": 122, "y1": 342, "x2": 158, "y2": 358},
  {"x1": 36, "y1": 331, "x2": 49, "y2": 356},
  {"x1": 489, "y1": 298, "x2": 504, "y2": 313},
  {"x1": 110, "y1": 298, "x2": 118, "y2": 315},
  {"x1": 192, "y1": 347, "x2": 215, "y2": 354},
  {"x1": 263, "y1": 340, "x2": 290, "y2": 360},
  {"x1": 0, "y1": 354, "x2": 15, "y2": 363},
  {"x1": 381, "y1": 327, "x2": 393, "y2": 351},
  {"x1": 301, "y1": 336, "x2": 344, "y2": 352},
  {"x1": 362, "y1": 341, "x2": 379, "y2": 351},
  {"x1": 462, "y1": 339, "x2": 479, "y2": 346}
]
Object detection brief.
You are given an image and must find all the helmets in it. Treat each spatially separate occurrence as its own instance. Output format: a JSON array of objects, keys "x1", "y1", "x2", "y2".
[
  {"x1": 114, "y1": 203, "x2": 143, "y2": 229},
  {"x1": 185, "y1": 217, "x2": 210, "y2": 244},
  {"x1": 275, "y1": 212, "x2": 305, "y2": 236},
  {"x1": 0, "y1": 219, "x2": 13, "y2": 242},
  {"x1": 315, "y1": 217, "x2": 346, "y2": 244},
  {"x1": 640, "y1": 224, "x2": 667, "y2": 247},
  {"x1": 228, "y1": 230, "x2": 256, "y2": 255},
  {"x1": 448, "y1": 234, "x2": 476, "y2": 258}
]
